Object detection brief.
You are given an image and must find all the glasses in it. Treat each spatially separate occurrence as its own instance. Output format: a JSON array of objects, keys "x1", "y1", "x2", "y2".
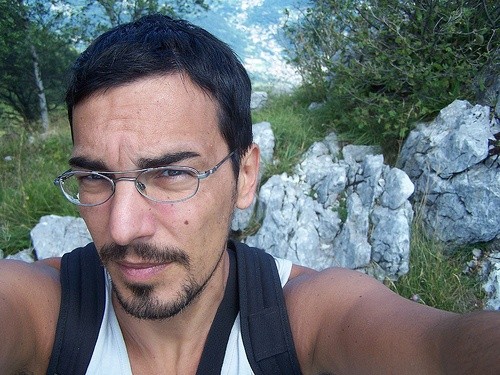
[{"x1": 51, "y1": 147, "x2": 240, "y2": 204}]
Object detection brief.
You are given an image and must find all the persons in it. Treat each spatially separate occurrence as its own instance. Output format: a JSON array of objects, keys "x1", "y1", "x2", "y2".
[{"x1": 0, "y1": 13, "x2": 500, "y2": 375}]
